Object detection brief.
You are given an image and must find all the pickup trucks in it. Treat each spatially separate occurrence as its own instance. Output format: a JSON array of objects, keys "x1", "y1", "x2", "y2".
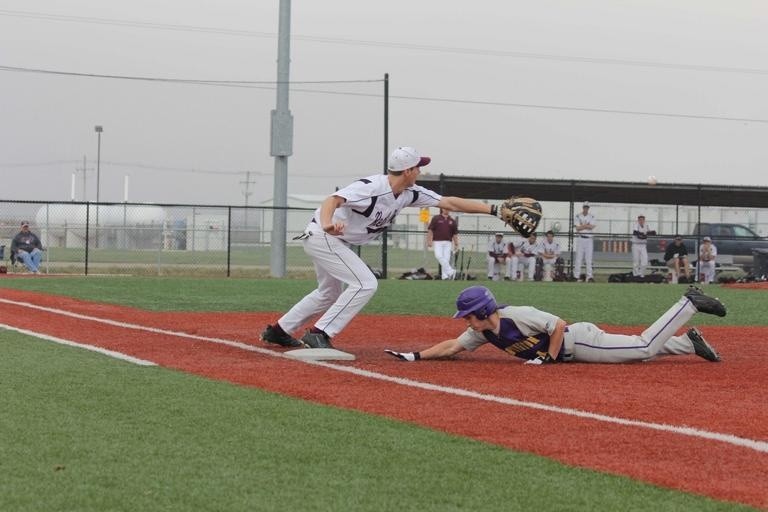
[{"x1": 645, "y1": 221, "x2": 767, "y2": 271}]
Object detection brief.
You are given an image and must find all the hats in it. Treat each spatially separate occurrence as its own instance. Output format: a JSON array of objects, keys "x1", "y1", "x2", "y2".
[
  {"x1": 388, "y1": 147, "x2": 431, "y2": 171},
  {"x1": 638, "y1": 216, "x2": 644, "y2": 218},
  {"x1": 496, "y1": 232, "x2": 504, "y2": 236},
  {"x1": 703, "y1": 237, "x2": 710, "y2": 241},
  {"x1": 21, "y1": 222, "x2": 28, "y2": 227},
  {"x1": 582, "y1": 201, "x2": 589, "y2": 208},
  {"x1": 675, "y1": 235, "x2": 683, "y2": 240}
]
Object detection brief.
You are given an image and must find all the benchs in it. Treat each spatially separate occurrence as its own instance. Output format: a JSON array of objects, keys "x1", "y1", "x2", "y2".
[{"x1": 489, "y1": 259, "x2": 740, "y2": 282}]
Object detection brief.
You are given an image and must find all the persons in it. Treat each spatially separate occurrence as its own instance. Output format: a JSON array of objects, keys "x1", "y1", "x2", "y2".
[
  {"x1": 572, "y1": 200, "x2": 596, "y2": 283},
  {"x1": 631, "y1": 215, "x2": 649, "y2": 277},
  {"x1": 508, "y1": 232, "x2": 538, "y2": 281},
  {"x1": 694, "y1": 236, "x2": 716, "y2": 284},
  {"x1": 426, "y1": 208, "x2": 460, "y2": 280},
  {"x1": 537, "y1": 231, "x2": 564, "y2": 281},
  {"x1": 486, "y1": 233, "x2": 510, "y2": 280},
  {"x1": 257, "y1": 147, "x2": 541, "y2": 348},
  {"x1": 664, "y1": 235, "x2": 691, "y2": 283},
  {"x1": 382, "y1": 284, "x2": 725, "y2": 365},
  {"x1": 11, "y1": 220, "x2": 44, "y2": 274}
]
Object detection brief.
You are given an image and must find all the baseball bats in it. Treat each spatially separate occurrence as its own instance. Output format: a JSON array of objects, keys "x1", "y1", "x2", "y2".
[
  {"x1": 460, "y1": 247, "x2": 464, "y2": 281},
  {"x1": 464, "y1": 244, "x2": 474, "y2": 281}
]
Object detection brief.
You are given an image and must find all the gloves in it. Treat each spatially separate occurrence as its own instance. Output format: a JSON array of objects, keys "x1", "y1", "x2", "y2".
[
  {"x1": 525, "y1": 353, "x2": 552, "y2": 365},
  {"x1": 385, "y1": 348, "x2": 420, "y2": 361}
]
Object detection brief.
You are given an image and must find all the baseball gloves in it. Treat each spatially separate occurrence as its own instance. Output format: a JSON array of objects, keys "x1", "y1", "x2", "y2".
[{"x1": 491, "y1": 198, "x2": 543, "y2": 238}]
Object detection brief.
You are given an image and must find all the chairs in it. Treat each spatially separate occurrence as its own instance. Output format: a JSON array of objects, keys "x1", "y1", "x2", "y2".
[{"x1": 10, "y1": 240, "x2": 43, "y2": 277}]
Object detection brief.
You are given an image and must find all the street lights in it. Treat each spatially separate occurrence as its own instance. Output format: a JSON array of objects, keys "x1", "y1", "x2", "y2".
[{"x1": 91, "y1": 124, "x2": 104, "y2": 250}]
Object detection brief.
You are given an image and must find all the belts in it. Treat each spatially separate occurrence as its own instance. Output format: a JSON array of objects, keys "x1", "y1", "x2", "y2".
[{"x1": 564, "y1": 327, "x2": 571, "y2": 358}]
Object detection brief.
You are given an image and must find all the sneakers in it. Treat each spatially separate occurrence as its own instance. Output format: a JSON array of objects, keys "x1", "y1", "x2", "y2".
[
  {"x1": 685, "y1": 285, "x2": 725, "y2": 318},
  {"x1": 299, "y1": 328, "x2": 335, "y2": 348},
  {"x1": 688, "y1": 327, "x2": 721, "y2": 362},
  {"x1": 258, "y1": 325, "x2": 301, "y2": 346}
]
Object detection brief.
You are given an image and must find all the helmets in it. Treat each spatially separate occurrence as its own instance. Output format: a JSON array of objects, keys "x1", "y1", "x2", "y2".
[{"x1": 451, "y1": 285, "x2": 498, "y2": 320}]
